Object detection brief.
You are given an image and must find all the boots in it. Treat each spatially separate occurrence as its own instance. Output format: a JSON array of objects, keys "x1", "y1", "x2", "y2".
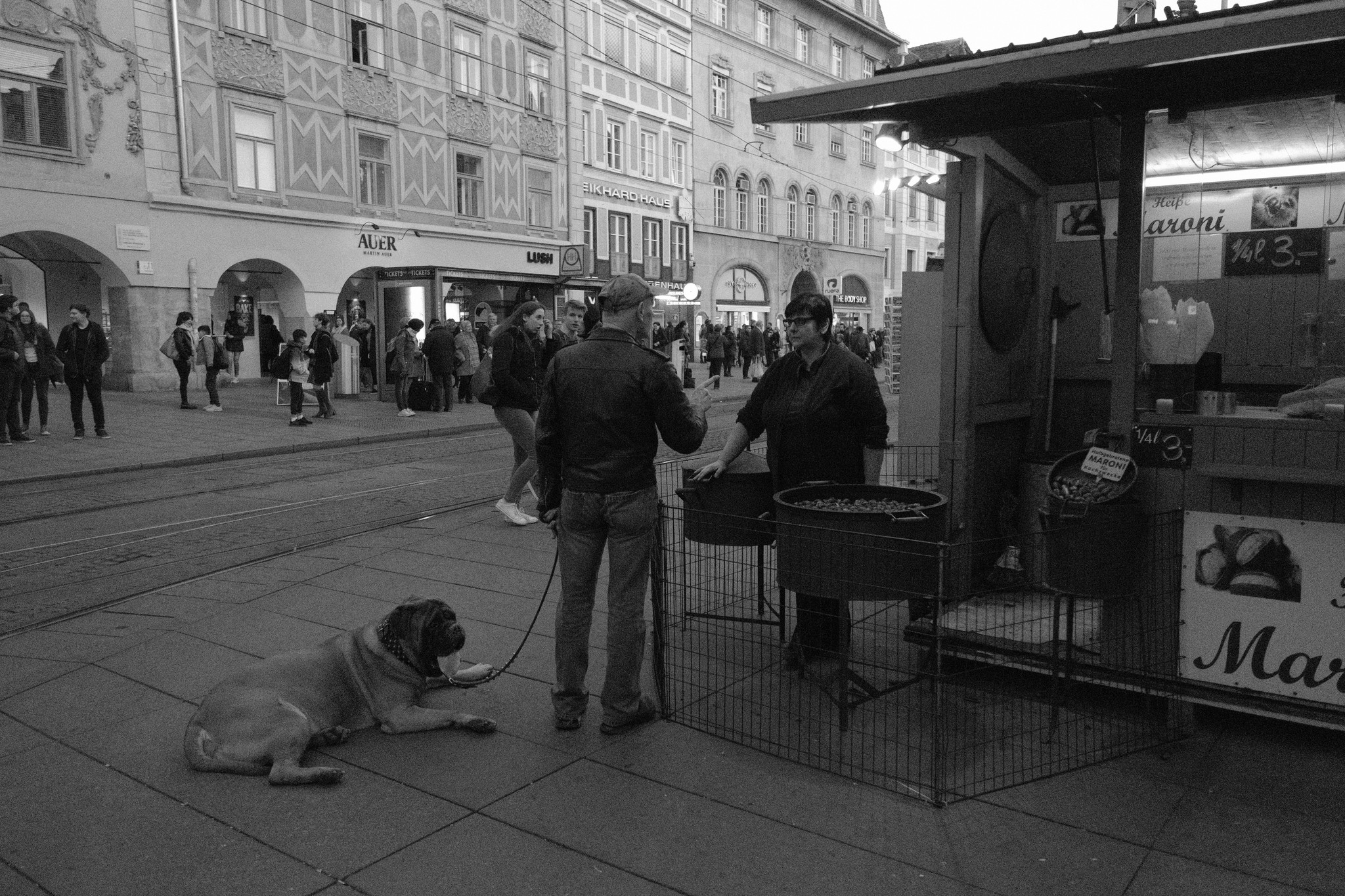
[{"x1": 311, "y1": 387, "x2": 337, "y2": 418}]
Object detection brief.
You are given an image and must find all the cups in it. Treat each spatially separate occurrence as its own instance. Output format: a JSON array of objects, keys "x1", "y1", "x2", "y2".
[{"x1": 1146, "y1": 351, "x2": 1238, "y2": 416}]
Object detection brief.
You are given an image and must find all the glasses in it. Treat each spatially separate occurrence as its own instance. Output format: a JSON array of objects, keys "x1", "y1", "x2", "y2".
[{"x1": 783, "y1": 317, "x2": 826, "y2": 327}]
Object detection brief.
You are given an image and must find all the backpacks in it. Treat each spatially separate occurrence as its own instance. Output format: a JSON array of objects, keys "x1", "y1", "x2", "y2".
[
  {"x1": 208, "y1": 337, "x2": 230, "y2": 369},
  {"x1": 270, "y1": 346, "x2": 292, "y2": 384}
]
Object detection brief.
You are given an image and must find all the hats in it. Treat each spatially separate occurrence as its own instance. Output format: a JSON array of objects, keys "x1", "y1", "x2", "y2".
[
  {"x1": 336, "y1": 314, "x2": 344, "y2": 321},
  {"x1": 428, "y1": 318, "x2": 441, "y2": 329},
  {"x1": 597, "y1": 272, "x2": 670, "y2": 313}
]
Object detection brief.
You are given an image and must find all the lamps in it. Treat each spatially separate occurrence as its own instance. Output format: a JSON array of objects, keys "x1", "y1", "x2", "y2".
[
  {"x1": 398, "y1": 228, "x2": 421, "y2": 240},
  {"x1": 355, "y1": 222, "x2": 379, "y2": 236},
  {"x1": 1145, "y1": 159, "x2": 1345, "y2": 188},
  {"x1": 875, "y1": 123, "x2": 910, "y2": 152}
]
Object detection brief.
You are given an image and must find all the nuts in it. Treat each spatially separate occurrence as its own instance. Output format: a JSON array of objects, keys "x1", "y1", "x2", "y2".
[
  {"x1": 1195, "y1": 521, "x2": 1301, "y2": 600},
  {"x1": 1052, "y1": 473, "x2": 1119, "y2": 503},
  {"x1": 791, "y1": 495, "x2": 924, "y2": 512}
]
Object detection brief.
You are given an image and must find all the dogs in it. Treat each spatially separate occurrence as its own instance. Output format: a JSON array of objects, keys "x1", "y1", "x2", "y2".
[{"x1": 184, "y1": 596, "x2": 494, "y2": 787}]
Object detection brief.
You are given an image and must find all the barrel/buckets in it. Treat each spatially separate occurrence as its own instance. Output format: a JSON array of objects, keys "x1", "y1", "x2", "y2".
[
  {"x1": 678, "y1": 448, "x2": 948, "y2": 600},
  {"x1": 1043, "y1": 448, "x2": 1141, "y2": 600}
]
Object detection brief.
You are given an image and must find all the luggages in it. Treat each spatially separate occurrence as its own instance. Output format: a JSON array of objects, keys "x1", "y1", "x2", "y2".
[{"x1": 408, "y1": 378, "x2": 434, "y2": 412}]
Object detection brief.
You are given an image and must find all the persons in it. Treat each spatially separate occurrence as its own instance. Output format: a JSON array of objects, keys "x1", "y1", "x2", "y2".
[
  {"x1": 691, "y1": 292, "x2": 891, "y2": 682},
  {"x1": 489, "y1": 301, "x2": 549, "y2": 524},
  {"x1": 527, "y1": 299, "x2": 586, "y2": 502},
  {"x1": 533, "y1": 273, "x2": 720, "y2": 738},
  {"x1": 1, "y1": 279, "x2": 888, "y2": 445}
]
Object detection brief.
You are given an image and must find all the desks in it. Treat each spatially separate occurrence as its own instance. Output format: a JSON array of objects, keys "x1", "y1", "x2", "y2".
[{"x1": 1033, "y1": 582, "x2": 1171, "y2": 760}]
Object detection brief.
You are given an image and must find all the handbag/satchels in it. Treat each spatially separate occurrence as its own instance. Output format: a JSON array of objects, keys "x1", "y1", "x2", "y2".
[
  {"x1": 747, "y1": 356, "x2": 764, "y2": 378},
  {"x1": 684, "y1": 368, "x2": 692, "y2": 379},
  {"x1": 706, "y1": 348, "x2": 711, "y2": 362},
  {"x1": 159, "y1": 330, "x2": 181, "y2": 361},
  {"x1": 683, "y1": 377, "x2": 696, "y2": 388},
  {"x1": 468, "y1": 348, "x2": 504, "y2": 406},
  {"x1": 48, "y1": 355, "x2": 64, "y2": 385}
]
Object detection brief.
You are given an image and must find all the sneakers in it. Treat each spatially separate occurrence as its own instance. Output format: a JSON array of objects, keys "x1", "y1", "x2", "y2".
[
  {"x1": 0, "y1": 377, "x2": 476, "y2": 447},
  {"x1": 527, "y1": 474, "x2": 542, "y2": 501},
  {"x1": 503, "y1": 507, "x2": 538, "y2": 524},
  {"x1": 554, "y1": 707, "x2": 585, "y2": 729},
  {"x1": 600, "y1": 698, "x2": 657, "y2": 735},
  {"x1": 699, "y1": 358, "x2": 772, "y2": 389},
  {"x1": 495, "y1": 498, "x2": 527, "y2": 525}
]
